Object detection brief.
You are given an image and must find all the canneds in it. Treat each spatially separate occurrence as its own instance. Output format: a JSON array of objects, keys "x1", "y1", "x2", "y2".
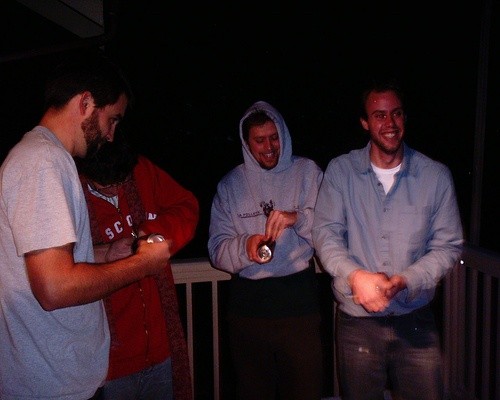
[
  {"x1": 130, "y1": 233, "x2": 165, "y2": 254},
  {"x1": 256, "y1": 236, "x2": 276, "y2": 262}
]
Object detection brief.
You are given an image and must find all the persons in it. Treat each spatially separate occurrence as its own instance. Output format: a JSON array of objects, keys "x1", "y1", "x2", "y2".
[
  {"x1": 208, "y1": 102, "x2": 326, "y2": 400},
  {"x1": 323, "y1": 81, "x2": 464, "y2": 400},
  {"x1": 75, "y1": 128, "x2": 199, "y2": 400},
  {"x1": 0, "y1": 53, "x2": 171, "y2": 400}
]
